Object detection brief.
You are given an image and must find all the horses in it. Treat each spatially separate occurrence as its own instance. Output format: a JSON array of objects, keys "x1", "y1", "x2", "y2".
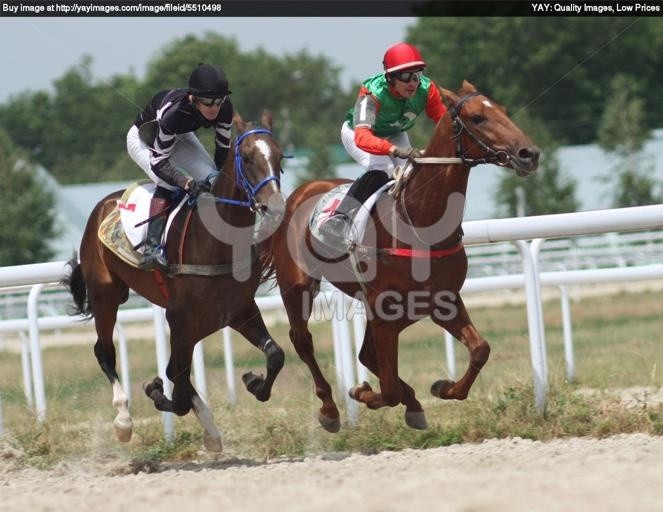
[
  {"x1": 254, "y1": 78, "x2": 540, "y2": 434},
  {"x1": 57, "y1": 112, "x2": 288, "y2": 457}
]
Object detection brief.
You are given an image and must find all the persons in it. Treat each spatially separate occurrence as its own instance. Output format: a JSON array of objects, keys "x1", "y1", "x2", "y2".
[
  {"x1": 126, "y1": 61, "x2": 233, "y2": 281},
  {"x1": 317, "y1": 41, "x2": 447, "y2": 236}
]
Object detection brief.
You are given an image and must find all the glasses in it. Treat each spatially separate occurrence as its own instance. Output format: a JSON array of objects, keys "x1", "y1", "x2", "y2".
[
  {"x1": 192, "y1": 94, "x2": 227, "y2": 107},
  {"x1": 388, "y1": 67, "x2": 423, "y2": 83}
]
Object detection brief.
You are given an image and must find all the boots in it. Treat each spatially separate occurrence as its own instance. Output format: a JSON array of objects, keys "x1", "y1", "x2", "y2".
[
  {"x1": 317, "y1": 175, "x2": 368, "y2": 244},
  {"x1": 137, "y1": 197, "x2": 175, "y2": 268}
]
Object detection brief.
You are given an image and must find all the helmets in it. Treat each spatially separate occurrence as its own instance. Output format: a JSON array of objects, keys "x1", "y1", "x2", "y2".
[{"x1": 380, "y1": 41, "x2": 427, "y2": 75}]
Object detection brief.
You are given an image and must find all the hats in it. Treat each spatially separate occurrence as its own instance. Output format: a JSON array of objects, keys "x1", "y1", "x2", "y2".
[{"x1": 186, "y1": 62, "x2": 233, "y2": 98}]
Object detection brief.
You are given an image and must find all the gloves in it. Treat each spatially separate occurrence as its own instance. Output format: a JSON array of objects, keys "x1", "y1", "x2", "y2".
[
  {"x1": 185, "y1": 179, "x2": 211, "y2": 198},
  {"x1": 391, "y1": 145, "x2": 423, "y2": 167}
]
